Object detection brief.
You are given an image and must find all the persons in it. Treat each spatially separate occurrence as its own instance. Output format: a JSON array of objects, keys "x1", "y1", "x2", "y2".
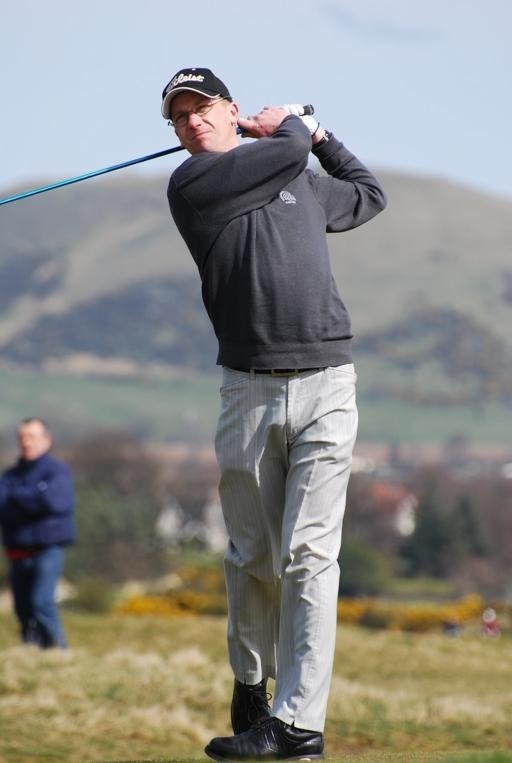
[
  {"x1": 0, "y1": 416, "x2": 79, "y2": 649},
  {"x1": 160, "y1": 67, "x2": 389, "y2": 763}
]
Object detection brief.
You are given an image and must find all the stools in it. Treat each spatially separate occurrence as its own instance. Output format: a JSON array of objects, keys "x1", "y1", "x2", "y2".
[{"x1": 229, "y1": 366, "x2": 319, "y2": 374}]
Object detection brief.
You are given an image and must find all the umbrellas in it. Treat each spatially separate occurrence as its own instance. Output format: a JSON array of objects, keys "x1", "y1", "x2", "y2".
[
  {"x1": 203, "y1": 716, "x2": 325, "y2": 763},
  {"x1": 230, "y1": 678, "x2": 272, "y2": 736}
]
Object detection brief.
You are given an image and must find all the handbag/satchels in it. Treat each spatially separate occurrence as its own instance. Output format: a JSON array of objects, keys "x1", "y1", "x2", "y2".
[
  {"x1": 311, "y1": 122, "x2": 320, "y2": 136},
  {"x1": 312, "y1": 129, "x2": 330, "y2": 149}
]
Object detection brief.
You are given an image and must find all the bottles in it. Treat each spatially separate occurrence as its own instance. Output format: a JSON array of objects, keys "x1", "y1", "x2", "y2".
[{"x1": 161, "y1": 67, "x2": 233, "y2": 120}]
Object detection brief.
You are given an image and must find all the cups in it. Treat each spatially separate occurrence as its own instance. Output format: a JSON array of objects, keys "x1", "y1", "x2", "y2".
[{"x1": 168, "y1": 98, "x2": 232, "y2": 128}]
